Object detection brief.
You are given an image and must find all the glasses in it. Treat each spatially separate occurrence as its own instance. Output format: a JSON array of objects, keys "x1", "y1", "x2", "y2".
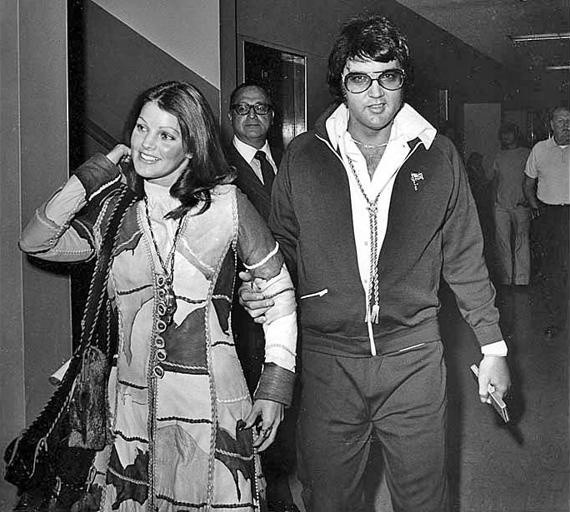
[
  {"x1": 229, "y1": 101, "x2": 275, "y2": 115},
  {"x1": 340, "y1": 70, "x2": 408, "y2": 94}
]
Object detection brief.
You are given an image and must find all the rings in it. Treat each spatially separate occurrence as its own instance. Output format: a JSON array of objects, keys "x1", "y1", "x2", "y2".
[{"x1": 260, "y1": 426, "x2": 274, "y2": 439}]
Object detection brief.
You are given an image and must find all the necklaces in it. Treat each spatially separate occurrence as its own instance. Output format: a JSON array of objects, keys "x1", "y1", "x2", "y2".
[{"x1": 350, "y1": 134, "x2": 389, "y2": 159}]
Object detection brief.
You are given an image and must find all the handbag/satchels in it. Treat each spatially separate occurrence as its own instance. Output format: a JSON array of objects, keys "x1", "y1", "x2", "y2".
[{"x1": 2, "y1": 343, "x2": 110, "y2": 496}]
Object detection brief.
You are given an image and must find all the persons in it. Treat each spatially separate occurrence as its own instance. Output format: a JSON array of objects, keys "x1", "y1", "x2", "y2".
[
  {"x1": 237, "y1": 11, "x2": 521, "y2": 512},
  {"x1": 225, "y1": 81, "x2": 296, "y2": 477},
  {"x1": 463, "y1": 151, "x2": 494, "y2": 201},
  {"x1": 522, "y1": 105, "x2": 570, "y2": 288},
  {"x1": 17, "y1": 78, "x2": 299, "y2": 512},
  {"x1": 486, "y1": 122, "x2": 532, "y2": 289}
]
